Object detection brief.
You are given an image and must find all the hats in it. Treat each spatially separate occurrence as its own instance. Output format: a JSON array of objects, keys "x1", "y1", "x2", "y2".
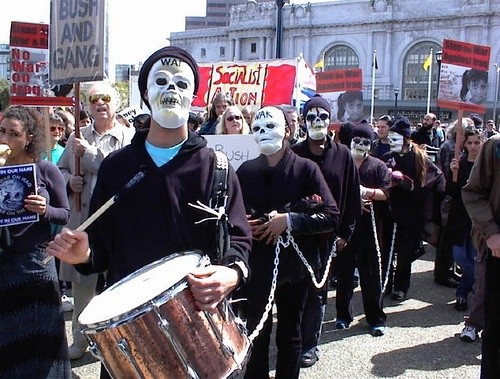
[
  {"x1": 272, "y1": 104, "x2": 295, "y2": 142},
  {"x1": 387, "y1": 118, "x2": 412, "y2": 138},
  {"x1": 135, "y1": 46, "x2": 200, "y2": 111},
  {"x1": 472, "y1": 116, "x2": 487, "y2": 127},
  {"x1": 302, "y1": 93, "x2": 332, "y2": 122},
  {"x1": 351, "y1": 123, "x2": 375, "y2": 142}
]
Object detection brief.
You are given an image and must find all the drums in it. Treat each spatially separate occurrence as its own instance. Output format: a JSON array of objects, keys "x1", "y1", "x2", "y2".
[{"x1": 77, "y1": 250, "x2": 254, "y2": 379}]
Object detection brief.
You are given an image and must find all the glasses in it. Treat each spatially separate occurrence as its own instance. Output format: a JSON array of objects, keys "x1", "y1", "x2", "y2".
[
  {"x1": 87, "y1": 94, "x2": 111, "y2": 103},
  {"x1": 50, "y1": 126, "x2": 64, "y2": 132}
]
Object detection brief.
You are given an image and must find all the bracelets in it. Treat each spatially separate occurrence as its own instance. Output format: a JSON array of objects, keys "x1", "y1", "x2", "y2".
[
  {"x1": 286, "y1": 212, "x2": 291, "y2": 229},
  {"x1": 368, "y1": 188, "x2": 375, "y2": 199}
]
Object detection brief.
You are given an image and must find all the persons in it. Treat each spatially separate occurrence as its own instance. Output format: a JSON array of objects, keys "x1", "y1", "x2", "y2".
[
  {"x1": 335, "y1": 89, "x2": 363, "y2": 124},
  {"x1": 0, "y1": 45, "x2": 500, "y2": 379},
  {"x1": 460, "y1": 68, "x2": 488, "y2": 106}
]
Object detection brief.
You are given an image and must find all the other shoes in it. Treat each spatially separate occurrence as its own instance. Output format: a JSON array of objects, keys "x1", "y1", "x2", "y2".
[
  {"x1": 461, "y1": 325, "x2": 481, "y2": 341},
  {"x1": 433, "y1": 275, "x2": 462, "y2": 288},
  {"x1": 370, "y1": 326, "x2": 386, "y2": 337},
  {"x1": 335, "y1": 320, "x2": 350, "y2": 329},
  {"x1": 390, "y1": 290, "x2": 405, "y2": 300},
  {"x1": 62, "y1": 296, "x2": 73, "y2": 310},
  {"x1": 68, "y1": 344, "x2": 84, "y2": 359},
  {"x1": 300, "y1": 353, "x2": 318, "y2": 367}
]
